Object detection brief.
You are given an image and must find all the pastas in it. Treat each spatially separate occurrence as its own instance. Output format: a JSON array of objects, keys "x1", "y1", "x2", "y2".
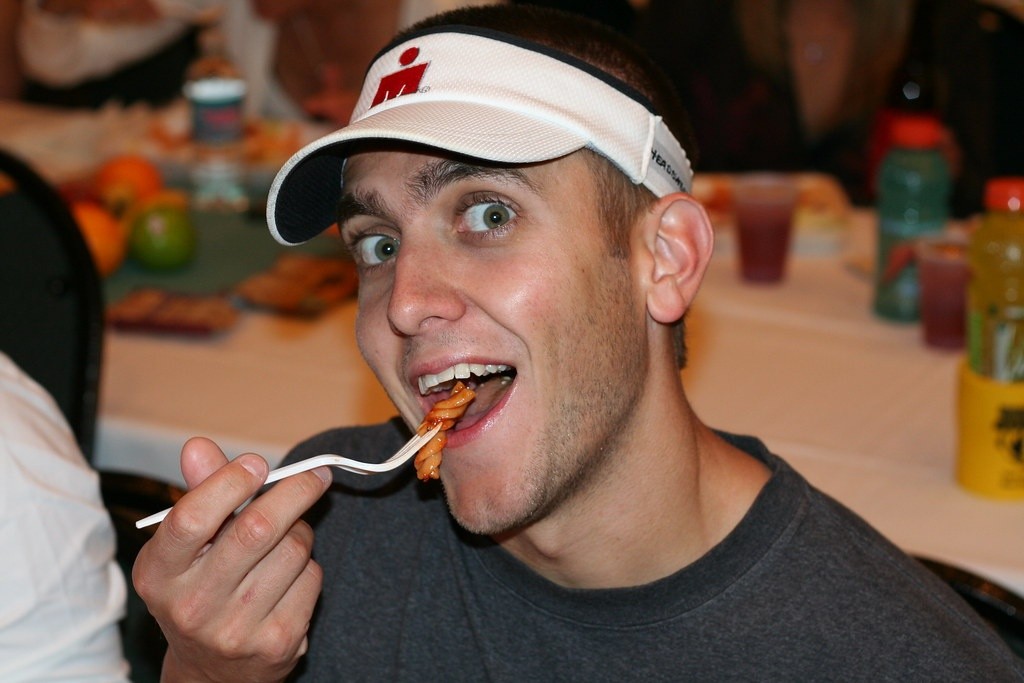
[{"x1": 414, "y1": 382, "x2": 476, "y2": 480}]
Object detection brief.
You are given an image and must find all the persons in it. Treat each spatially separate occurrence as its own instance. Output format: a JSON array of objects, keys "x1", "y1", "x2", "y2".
[
  {"x1": 132, "y1": 1, "x2": 1024, "y2": 683},
  {"x1": 0, "y1": 148, "x2": 129, "y2": 683}
]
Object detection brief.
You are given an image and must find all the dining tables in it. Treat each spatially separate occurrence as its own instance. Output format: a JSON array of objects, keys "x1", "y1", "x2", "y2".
[{"x1": 0, "y1": 103, "x2": 1023, "y2": 602}]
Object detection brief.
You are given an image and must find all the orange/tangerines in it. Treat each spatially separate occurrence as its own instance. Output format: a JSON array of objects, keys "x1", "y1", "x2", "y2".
[{"x1": 68, "y1": 151, "x2": 167, "y2": 279}]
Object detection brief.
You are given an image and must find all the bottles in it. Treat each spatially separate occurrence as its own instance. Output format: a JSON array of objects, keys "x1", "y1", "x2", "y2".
[
  {"x1": 182, "y1": 30, "x2": 251, "y2": 215},
  {"x1": 956, "y1": 180, "x2": 1024, "y2": 505},
  {"x1": 876, "y1": 117, "x2": 954, "y2": 323}
]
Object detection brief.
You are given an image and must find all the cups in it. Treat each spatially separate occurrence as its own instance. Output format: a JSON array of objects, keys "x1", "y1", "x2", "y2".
[
  {"x1": 920, "y1": 237, "x2": 965, "y2": 352},
  {"x1": 734, "y1": 179, "x2": 797, "y2": 286}
]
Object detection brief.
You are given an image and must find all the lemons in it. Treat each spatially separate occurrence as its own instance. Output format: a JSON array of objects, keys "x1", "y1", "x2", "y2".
[{"x1": 131, "y1": 202, "x2": 192, "y2": 270}]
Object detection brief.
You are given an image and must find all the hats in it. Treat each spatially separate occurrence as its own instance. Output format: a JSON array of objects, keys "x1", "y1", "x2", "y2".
[{"x1": 265, "y1": 32, "x2": 694, "y2": 248}]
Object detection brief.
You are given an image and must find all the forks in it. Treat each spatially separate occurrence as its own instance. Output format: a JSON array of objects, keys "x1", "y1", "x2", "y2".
[{"x1": 136, "y1": 422, "x2": 444, "y2": 529}]
